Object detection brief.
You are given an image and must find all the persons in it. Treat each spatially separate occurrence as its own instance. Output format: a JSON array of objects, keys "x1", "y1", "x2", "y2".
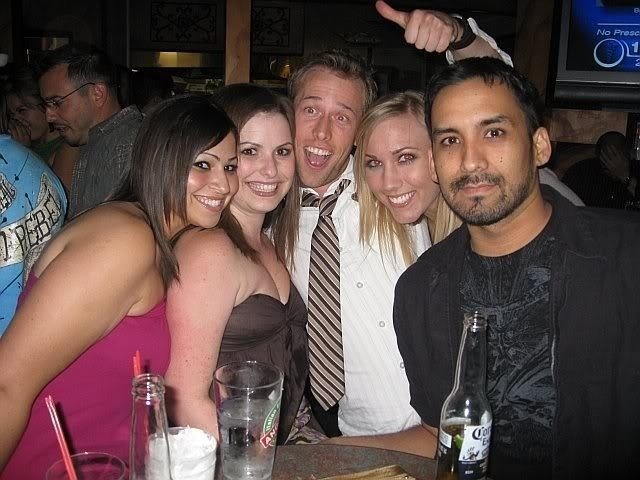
[{"x1": 1, "y1": 0, "x2": 640, "y2": 479}]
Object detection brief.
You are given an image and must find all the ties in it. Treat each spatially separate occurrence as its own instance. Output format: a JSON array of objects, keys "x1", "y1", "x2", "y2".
[{"x1": 300, "y1": 178, "x2": 351, "y2": 412}]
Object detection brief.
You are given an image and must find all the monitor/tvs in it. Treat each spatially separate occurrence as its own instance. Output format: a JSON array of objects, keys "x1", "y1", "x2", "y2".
[{"x1": 545, "y1": 0, "x2": 640, "y2": 112}]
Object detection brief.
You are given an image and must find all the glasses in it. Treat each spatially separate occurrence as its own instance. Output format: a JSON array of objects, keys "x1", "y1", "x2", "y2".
[{"x1": 37, "y1": 82, "x2": 96, "y2": 113}]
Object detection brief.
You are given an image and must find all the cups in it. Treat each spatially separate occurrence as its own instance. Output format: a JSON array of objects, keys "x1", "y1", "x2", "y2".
[
  {"x1": 143, "y1": 427, "x2": 219, "y2": 480},
  {"x1": 211, "y1": 362, "x2": 285, "y2": 476},
  {"x1": 45, "y1": 452, "x2": 125, "y2": 480}
]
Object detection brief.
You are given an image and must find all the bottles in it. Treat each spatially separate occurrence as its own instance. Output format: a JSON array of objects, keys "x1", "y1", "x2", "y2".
[
  {"x1": 436, "y1": 311, "x2": 492, "y2": 480},
  {"x1": 128, "y1": 374, "x2": 173, "y2": 479}
]
